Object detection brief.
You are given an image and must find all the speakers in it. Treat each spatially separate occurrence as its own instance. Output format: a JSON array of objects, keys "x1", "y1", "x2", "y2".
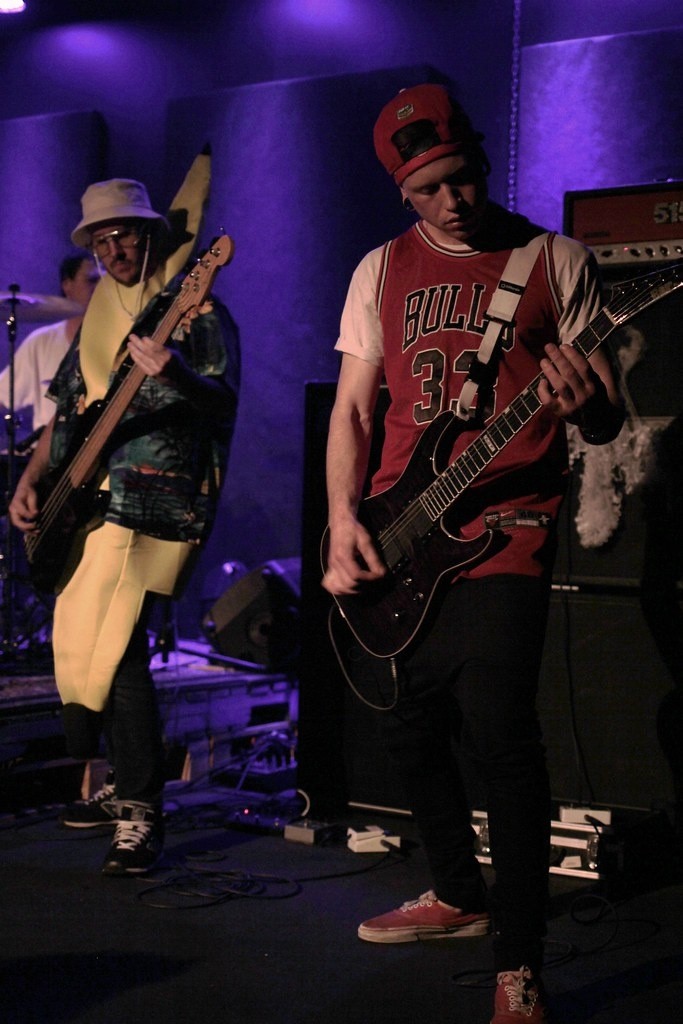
[
  {"x1": 203, "y1": 560, "x2": 302, "y2": 673},
  {"x1": 305, "y1": 260, "x2": 683, "y2": 814}
]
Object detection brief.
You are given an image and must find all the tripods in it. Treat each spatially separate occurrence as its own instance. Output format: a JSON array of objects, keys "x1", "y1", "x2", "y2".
[{"x1": 147, "y1": 599, "x2": 265, "y2": 672}]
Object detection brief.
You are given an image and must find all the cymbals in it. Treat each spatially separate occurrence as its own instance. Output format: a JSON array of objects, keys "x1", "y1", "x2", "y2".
[{"x1": 0, "y1": 289, "x2": 85, "y2": 323}]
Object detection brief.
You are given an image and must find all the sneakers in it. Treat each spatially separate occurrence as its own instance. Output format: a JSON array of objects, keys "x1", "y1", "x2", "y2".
[
  {"x1": 490, "y1": 964, "x2": 549, "y2": 1024},
  {"x1": 62, "y1": 768, "x2": 118, "y2": 827},
  {"x1": 101, "y1": 799, "x2": 164, "y2": 876},
  {"x1": 358, "y1": 890, "x2": 493, "y2": 944}
]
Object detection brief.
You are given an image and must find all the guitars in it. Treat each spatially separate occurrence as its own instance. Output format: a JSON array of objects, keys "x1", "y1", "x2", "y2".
[
  {"x1": 15, "y1": 224, "x2": 237, "y2": 600},
  {"x1": 318, "y1": 259, "x2": 683, "y2": 663}
]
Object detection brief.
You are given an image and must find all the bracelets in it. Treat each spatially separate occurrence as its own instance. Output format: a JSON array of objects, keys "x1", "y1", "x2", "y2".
[{"x1": 580, "y1": 406, "x2": 622, "y2": 441}]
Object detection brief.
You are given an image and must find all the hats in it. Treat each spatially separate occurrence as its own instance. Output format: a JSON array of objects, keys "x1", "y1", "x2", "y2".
[
  {"x1": 374, "y1": 82, "x2": 486, "y2": 188},
  {"x1": 70, "y1": 178, "x2": 172, "y2": 256}
]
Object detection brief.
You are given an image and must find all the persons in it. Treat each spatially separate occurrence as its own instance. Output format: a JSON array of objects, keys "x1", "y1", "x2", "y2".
[
  {"x1": 321, "y1": 84, "x2": 626, "y2": 1024},
  {"x1": 0, "y1": 254, "x2": 102, "y2": 450},
  {"x1": 8, "y1": 178, "x2": 241, "y2": 873}
]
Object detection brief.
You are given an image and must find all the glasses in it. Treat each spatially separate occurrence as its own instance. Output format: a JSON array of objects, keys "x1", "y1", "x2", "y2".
[{"x1": 91, "y1": 226, "x2": 144, "y2": 259}]
